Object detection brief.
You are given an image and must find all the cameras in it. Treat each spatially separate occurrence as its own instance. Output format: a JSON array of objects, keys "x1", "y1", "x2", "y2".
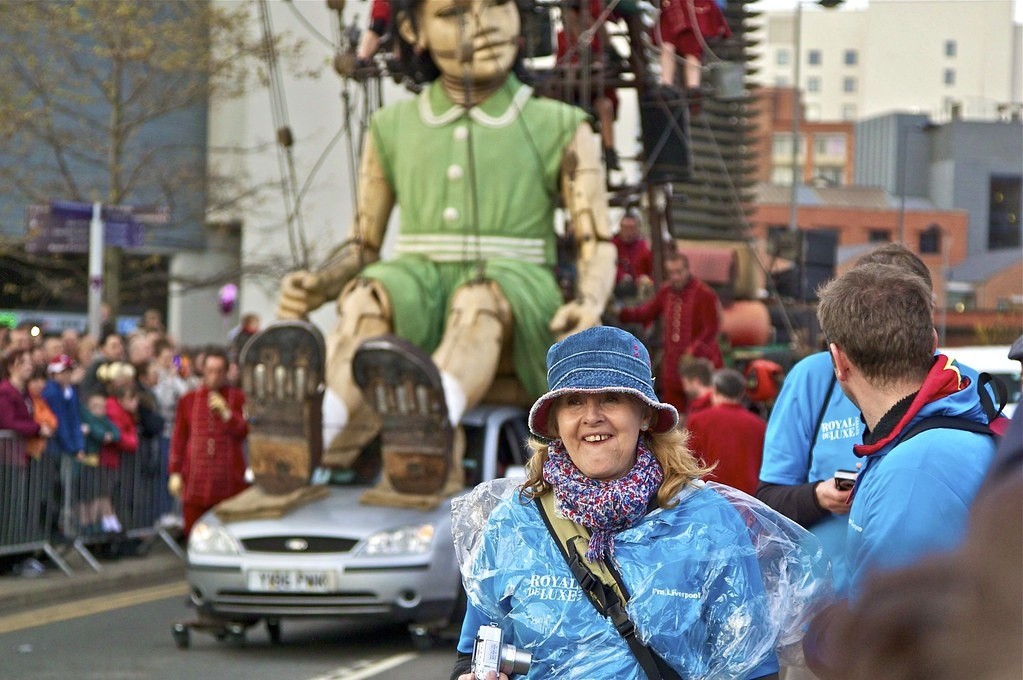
[
  {"x1": 473, "y1": 623, "x2": 532, "y2": 680},
  {"x1": 834, "y1": 471, "x2": 859, "y2": 491}
]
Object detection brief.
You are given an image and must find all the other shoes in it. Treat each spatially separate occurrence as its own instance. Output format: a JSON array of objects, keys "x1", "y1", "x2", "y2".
[{"x1": 102, "y1": 513, "x2": 122, "y2": 534}]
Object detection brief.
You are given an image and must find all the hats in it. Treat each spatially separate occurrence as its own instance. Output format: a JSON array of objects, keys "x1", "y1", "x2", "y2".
[
  {"x1": 46, "y1": 355, "x2": 75, "y2": 374},
  {"x1": 529, "y1": 326, "x2": 679, "y2": 440}
]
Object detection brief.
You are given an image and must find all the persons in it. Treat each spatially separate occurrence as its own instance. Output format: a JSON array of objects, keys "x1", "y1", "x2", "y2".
[
  {"x1": 0, "y1": 304, "x2": 259, "y2": 578},
  {"x1": 450, "y1": 326, "x2": 781, "y2": 680},
  {"x1": 167, "y1": 349, "x2": 250, "y2": 609},
  {"x1": 353, "y1": 0, "x2": 768, "y2": 497},
  {"x1": 238, "y1": 0, "x2": 620, "y2": 498},
  {"x1": 754, "y1": 242, "x2": 1023, "y2": 680}
]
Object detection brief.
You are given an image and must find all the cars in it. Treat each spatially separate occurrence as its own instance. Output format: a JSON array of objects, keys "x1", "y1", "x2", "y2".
[
  {"x1": 937, "y1": 342, "x2": 1022, "y2": 421},
  {"x1": 184, "y1": 401, "x2": 535, "y2": 628}
]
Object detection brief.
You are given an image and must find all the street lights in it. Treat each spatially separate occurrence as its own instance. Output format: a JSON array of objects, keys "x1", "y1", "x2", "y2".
[
  {"x1": 789, "y1": 0, "x2": 847, "y2": 302},
  {"x1": 891, "y1": 123, "x2": 942, "y2": 247}
]
